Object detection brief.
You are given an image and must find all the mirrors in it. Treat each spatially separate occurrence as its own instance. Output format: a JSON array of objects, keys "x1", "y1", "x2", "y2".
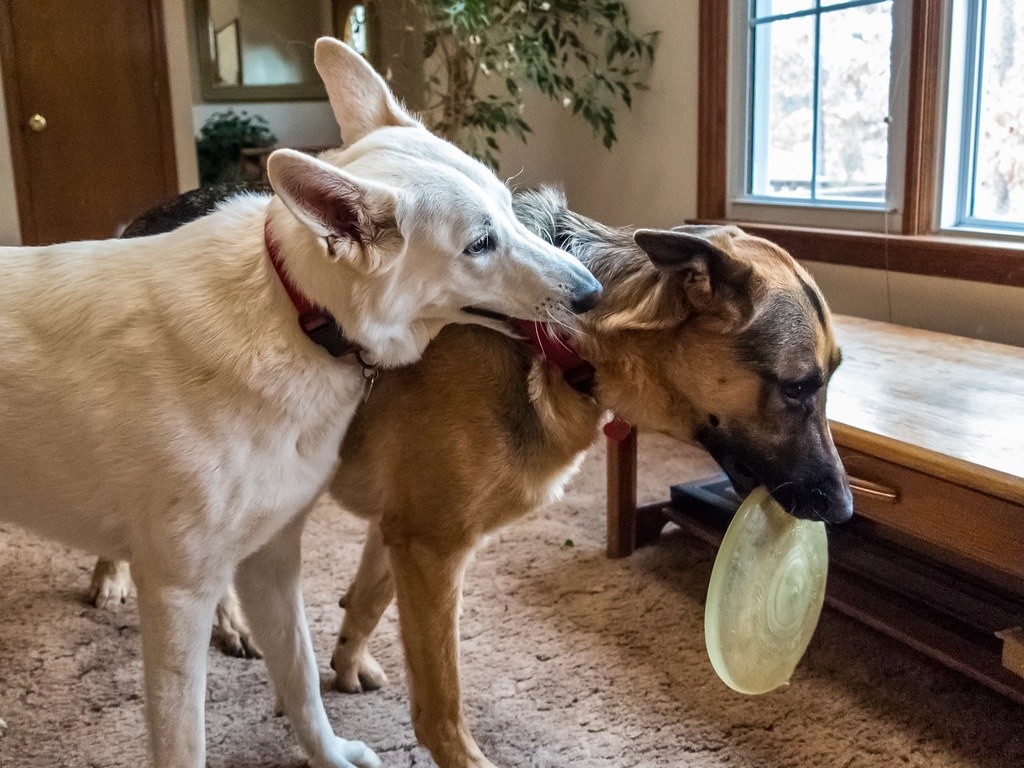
[{"x1": 197, "y1": 1, "x2": 381, "y2": 104}]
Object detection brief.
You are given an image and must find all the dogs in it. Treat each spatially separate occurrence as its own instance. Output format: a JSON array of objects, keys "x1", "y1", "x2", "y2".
[
  {"x1": 83, "y1": 176, "x2": 857, "y2": 768},
  {"x1": 1, "y1": 33, "x2": 604, "y2": 768}
]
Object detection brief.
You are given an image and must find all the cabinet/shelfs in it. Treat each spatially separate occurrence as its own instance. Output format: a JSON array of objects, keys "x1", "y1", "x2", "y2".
[{"x1": 608, "y1": 310, "x2": 1024, "y2": 701}]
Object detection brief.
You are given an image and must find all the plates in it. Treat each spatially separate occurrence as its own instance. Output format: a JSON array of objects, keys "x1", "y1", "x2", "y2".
[{"x1": 703, "y1": 484, "x2": 829, "y2": 696}]
